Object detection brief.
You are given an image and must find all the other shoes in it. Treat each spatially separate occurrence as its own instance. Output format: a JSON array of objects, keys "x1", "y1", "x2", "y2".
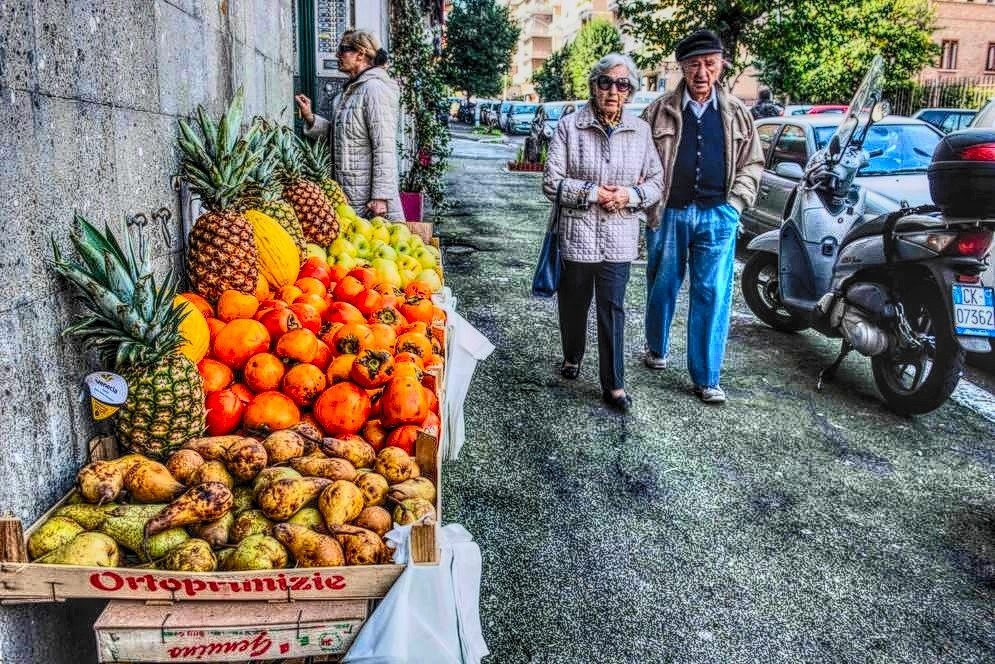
[
  {"x1": 561, "y1": 358, "x2": 580, "y2": 379},
  {"x1": 645, "y1": 350, "x2": 667, "y2": 369},
  {"x1": 696, "y1": 383, "x2": 726, "y2": 401},
  {"x1": 603, "y1": 389, "x2": 632, "y2": 409}
]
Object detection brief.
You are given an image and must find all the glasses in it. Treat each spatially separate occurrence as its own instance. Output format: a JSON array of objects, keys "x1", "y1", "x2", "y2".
[
  {"x1": 590, "y1": 73, "x2": 632, "y2": 94},
  {"x1": 338, "y1": 43, "x2": 356, "y2": 53}
]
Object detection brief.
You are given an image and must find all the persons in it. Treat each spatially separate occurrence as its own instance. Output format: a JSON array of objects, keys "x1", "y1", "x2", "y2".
[
  {"x1": 749, "y1": 85, "x2": 785, "y2": 118},
  {"x1": 540, "y1": 52, "x2": 669, "y2": 409},
  {"x1": 293, "y1": 27, "x2": 409, "y2": 228},
  {"x1": 636, "y1": 29, "x2": 765, "y2": 401}
]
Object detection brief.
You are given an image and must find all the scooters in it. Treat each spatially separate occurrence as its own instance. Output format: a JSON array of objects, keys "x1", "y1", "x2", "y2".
[{"x1": 740, "y1": 54, "x2": 995, "y2": 417}]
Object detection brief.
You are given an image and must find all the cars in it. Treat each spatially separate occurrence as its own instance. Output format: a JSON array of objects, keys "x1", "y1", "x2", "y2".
[
  {"x1": 738, "y1": 99, "x2": 995, "y2": 271},
  {"x1": 456, "y1": 93, "x2": 670, "y2": 163}
]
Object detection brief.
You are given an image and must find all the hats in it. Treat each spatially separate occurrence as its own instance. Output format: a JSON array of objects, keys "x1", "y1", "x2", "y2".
[{"x1": 676, "y1": 29, "x2": 724, "y2": 61}]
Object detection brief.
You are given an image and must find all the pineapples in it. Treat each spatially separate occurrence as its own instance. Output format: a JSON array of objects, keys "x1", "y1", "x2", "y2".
[{"x1": 41, "y1": 88, "x2": 350, "y2": 468}]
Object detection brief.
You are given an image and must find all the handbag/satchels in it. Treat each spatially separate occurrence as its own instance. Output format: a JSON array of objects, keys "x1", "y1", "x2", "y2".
[{"x1": 532, "y1": 179, "x2": 567, "y2": 298}]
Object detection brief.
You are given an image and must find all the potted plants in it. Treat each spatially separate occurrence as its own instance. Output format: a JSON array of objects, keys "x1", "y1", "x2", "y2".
[{"x1": 400, "y1": 158, "x2": 450, "y2": 222}]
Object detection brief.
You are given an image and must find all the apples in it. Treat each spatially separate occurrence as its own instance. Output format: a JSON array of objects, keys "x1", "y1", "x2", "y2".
[{"x1": 323, "y1": 211, "x2": 442, "y2": 296}]
